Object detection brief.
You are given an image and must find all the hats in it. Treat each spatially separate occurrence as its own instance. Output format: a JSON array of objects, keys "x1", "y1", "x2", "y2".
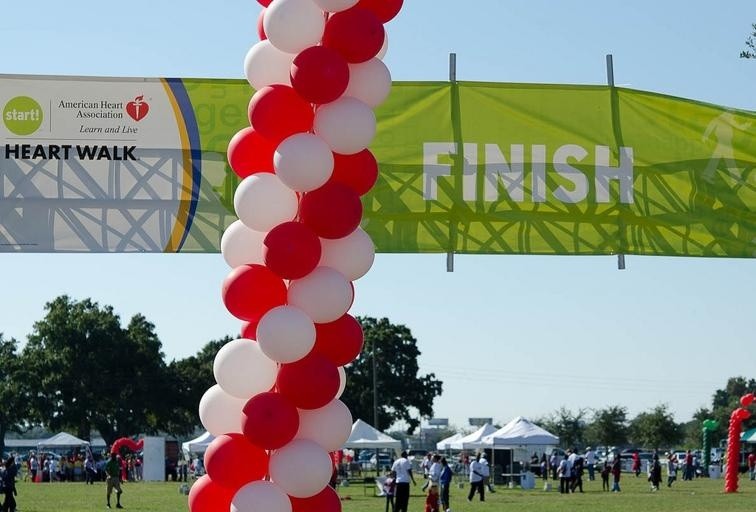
[{"x1": 480, "y1": 452, "x2": 487, "y2": 458}]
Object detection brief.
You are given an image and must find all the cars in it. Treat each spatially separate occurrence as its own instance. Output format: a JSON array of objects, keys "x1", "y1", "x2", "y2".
[
  {"x1": 0, "y1": 449, "x2": 204, "y2": 472},
  {"x1": 551, "y1": 438, "x2": 752, "y2": 479},
  {"x1": 337, "y1": 448, "x2": 477, "y2": 474}
]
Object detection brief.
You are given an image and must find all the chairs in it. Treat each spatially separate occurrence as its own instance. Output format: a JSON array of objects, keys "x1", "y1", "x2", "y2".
[{"x1": 360, "y1": 475, "x2": 377, "y2": 495}]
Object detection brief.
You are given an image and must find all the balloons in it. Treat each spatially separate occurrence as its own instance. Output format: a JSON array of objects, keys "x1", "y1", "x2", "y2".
[
  {"x1": 700, "y1": 417, "x2": 720, "y2": 471},
  {"x1": 189, "y1": 220, "x2": 376, "y2": 512},
  {"x1": 226, "y1": 1, "x2": 402, "y2": 239},
  {"x1": 725, "y1": 394, "x2": 754, "y2": 492}
]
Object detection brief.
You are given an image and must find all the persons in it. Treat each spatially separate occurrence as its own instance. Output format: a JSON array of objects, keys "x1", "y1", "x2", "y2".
[{"x1": 0, "y1": 443, "x2": 754, "y2": 512}]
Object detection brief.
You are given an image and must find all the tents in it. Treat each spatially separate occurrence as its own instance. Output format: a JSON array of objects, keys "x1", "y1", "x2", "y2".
[
  {"x1": 36, "y1": 432, "x2": 91, "y2": 462},
  {"x1": 437, "y1": 417, "x2": 560, "y2": 486},
  {"x1": 182, "y1": 431, "x2": 217, "y2": 460},
  {"x1": 334, "y1": 418, "x2": 402, "y2": 460}
]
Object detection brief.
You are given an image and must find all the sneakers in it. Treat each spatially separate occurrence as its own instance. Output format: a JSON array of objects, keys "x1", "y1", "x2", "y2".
[
  {"x1": 116, "y1": 503, "x2": 123, "y2": 509},
  {"x1": 106, "y1": 503, "x2": 112, "y2": 509}
]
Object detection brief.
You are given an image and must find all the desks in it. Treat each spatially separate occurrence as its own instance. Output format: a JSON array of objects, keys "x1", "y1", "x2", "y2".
[{"x1": 501, "y1": 470, "x2": 527, "y2": 488}]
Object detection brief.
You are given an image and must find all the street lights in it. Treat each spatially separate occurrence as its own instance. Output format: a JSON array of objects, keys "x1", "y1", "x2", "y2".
[{"x1": 369, "y1": 342, "x2": 383, "y2": 477}]
